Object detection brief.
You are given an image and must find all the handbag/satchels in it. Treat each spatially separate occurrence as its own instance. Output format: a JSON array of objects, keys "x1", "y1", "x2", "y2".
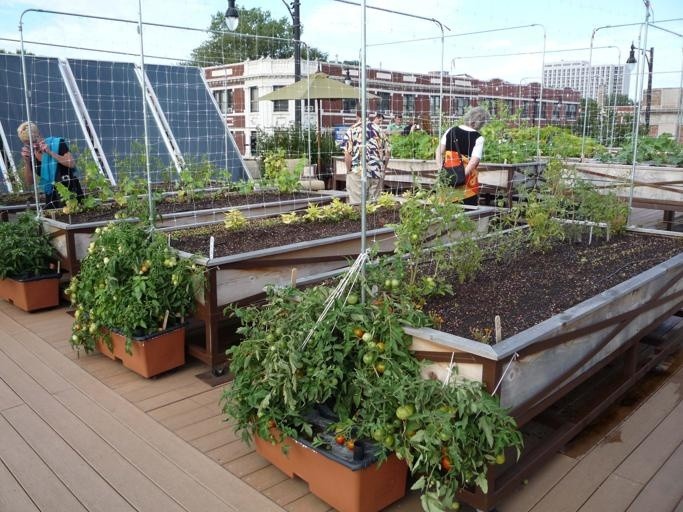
[{"x1": 440, "y1": 127, "x2": 466, "y2": 187}]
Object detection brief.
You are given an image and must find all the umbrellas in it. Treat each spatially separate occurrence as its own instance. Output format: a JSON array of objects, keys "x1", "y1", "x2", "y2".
[{"x1": 250, "y1": 73, "x2": 381, "y2": 179}]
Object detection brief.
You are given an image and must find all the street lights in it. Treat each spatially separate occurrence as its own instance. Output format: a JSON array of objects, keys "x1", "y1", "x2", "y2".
[
  {"x1": 223, "y1": 0, "x2": 301, "y2": 134},
  {"x1": 626, "y1": 40, "x2": 654, "y2": 135}
]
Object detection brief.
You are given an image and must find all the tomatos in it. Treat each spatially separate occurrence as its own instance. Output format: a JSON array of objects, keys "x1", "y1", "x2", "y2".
[
  {"x1": 256, "y1": 275, "x2": 506, "y2": 471},
  {"x1": 64, "y1": 213, "x2": 196, "y2": 344},
  {"x1": 452, "y1": 502, "x2": 461, "y2": 510}
]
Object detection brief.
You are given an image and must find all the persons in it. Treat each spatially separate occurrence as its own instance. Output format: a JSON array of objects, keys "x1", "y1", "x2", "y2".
[
  {"x1": 341, "y1": 108, "x2": 393, "y2": 207},
  {"x1": 371, "y1": 112, "x2": 434, "y2": 137},
  {"x1": 434, "y1": 106, "x2": 491, "y2": 212},
  {"x1": 14, "y1": 120, "x2": 85, "y2": 214}
]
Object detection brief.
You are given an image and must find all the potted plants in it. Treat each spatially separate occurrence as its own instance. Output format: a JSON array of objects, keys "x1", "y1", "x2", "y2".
[
  {"x1": 63, "y1": 213, "x2": 204, "y2": 379},
  {"x1": 0, "y1": 209, "x2": 62, "y2": 312},
  {"x1": 218, "y1": 251, "x2": 526, "y2": 512}
]
